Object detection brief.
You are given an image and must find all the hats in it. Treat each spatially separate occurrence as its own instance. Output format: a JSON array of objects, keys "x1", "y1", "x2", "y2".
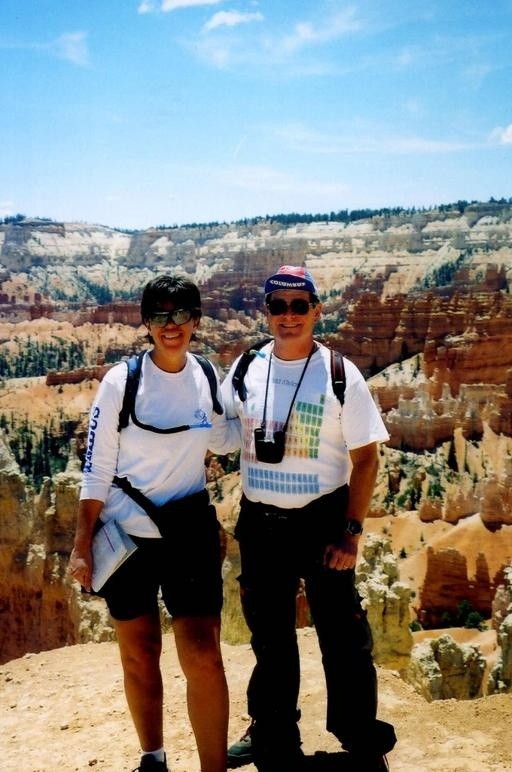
[{"x1": 264, "y1": 265, "x2": 316, "y2": 298}]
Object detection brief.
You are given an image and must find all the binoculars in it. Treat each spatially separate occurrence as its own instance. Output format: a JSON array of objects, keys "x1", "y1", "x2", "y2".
[{"x1": 254, "y1": 428, "x2": 286, "y2": 464}]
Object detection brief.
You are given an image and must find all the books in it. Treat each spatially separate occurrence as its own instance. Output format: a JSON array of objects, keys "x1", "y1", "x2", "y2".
[{"x1": 87, "y1": 517, "x2": 137, "y2": 594}]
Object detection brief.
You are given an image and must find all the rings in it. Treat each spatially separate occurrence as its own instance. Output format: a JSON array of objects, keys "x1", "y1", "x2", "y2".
[{"x1": 343, "y1": 564, "x2": 349, "y2": 569}]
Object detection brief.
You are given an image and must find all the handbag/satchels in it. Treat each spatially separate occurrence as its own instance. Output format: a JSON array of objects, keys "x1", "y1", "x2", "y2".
[{"x1": 147, "y1": 488, "x2": 223, "y2": 568}]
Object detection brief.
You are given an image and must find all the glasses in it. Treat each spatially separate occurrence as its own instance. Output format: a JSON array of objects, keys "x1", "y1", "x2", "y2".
[
  {"x1": 267, "y1": 299, "x2": 314, "y2": 316},
  {"x1": 147, "y1": 306, "x2": 200, "y2": 328}
]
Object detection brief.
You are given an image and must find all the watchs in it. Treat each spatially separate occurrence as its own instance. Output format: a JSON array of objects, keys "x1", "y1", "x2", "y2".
[{"x1": 341, "y1": 518, "x2": 366, "y2": 537}]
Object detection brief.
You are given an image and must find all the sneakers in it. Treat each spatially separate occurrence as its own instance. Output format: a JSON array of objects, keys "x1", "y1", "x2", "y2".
[
  {"x1": 225, "y1": 723, "x2": 302, "y2": 768},
  {"x1": 139, "y1": 752, "x2": 169, "y2": 772}
]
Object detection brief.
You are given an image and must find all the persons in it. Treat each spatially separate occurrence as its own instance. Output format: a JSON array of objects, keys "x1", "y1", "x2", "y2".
[
  {"x1": 65, "y1": 272, "x2": 247, "y2": 772},
  {"x1": 218, "y1": 261, "x2": 399, "y2": 771}
]
